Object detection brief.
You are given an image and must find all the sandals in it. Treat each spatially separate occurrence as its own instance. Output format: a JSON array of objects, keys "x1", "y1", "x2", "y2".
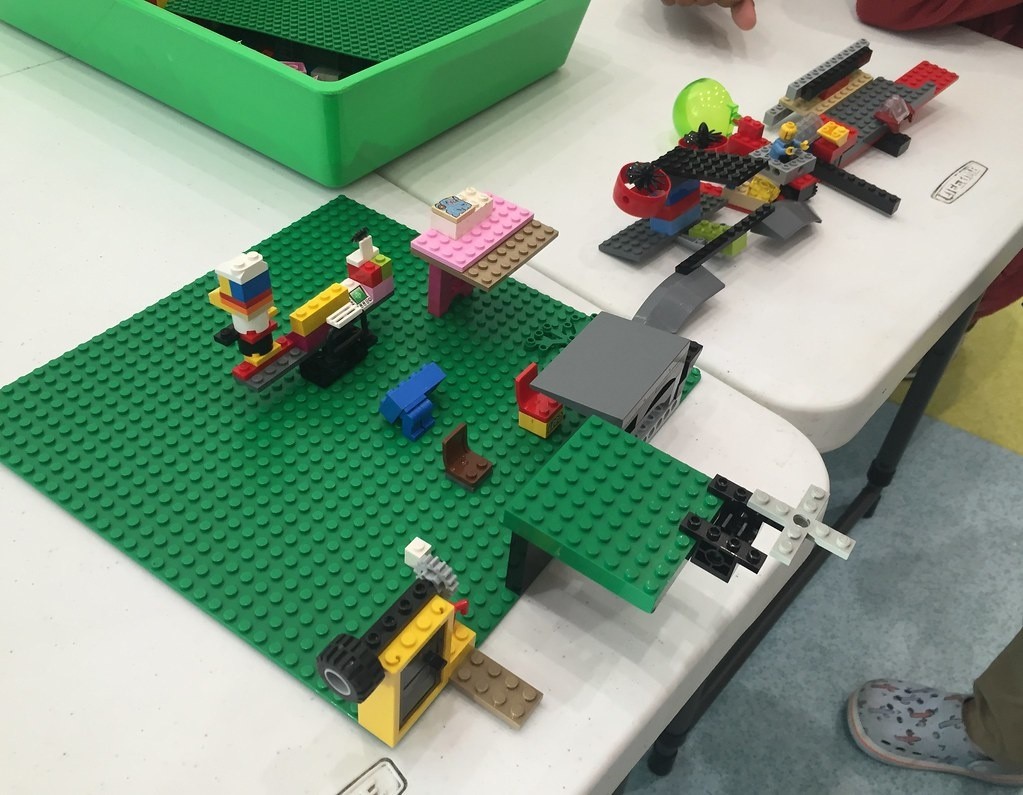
[{"x1": 847, "y1": 677, "x2": 1023, "y2": 789}]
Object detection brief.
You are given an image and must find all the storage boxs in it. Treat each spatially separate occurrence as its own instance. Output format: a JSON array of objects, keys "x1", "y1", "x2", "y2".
[{"x1": 0, "y1": 0, "x2": 589, "y2": 189}]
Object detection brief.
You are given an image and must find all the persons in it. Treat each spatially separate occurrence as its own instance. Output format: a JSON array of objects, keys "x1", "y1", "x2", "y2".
[
  {"x1": 848, "y1": 625, "x2": 1023, "y2": 786},
  {"x1": 661, "y1": 0, "x2": 1023, "y2": 332}
]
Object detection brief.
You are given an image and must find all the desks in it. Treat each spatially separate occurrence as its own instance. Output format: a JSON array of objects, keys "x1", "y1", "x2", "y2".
[{"x1": 1, "y1": 0, "x2": 1023, "y2": 795}]
